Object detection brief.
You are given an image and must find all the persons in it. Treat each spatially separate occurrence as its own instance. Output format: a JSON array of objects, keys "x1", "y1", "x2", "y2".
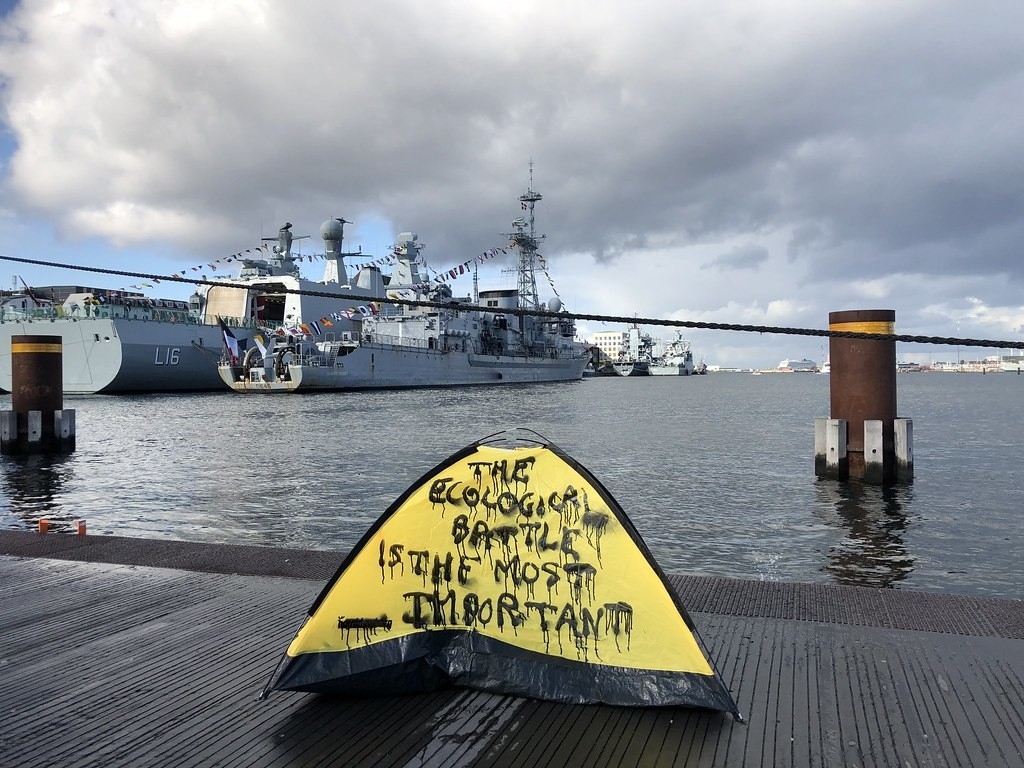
[
  {"x1": 193, "y1": 310, "x2": 198, "y2": 323},
  {"x1": 252, "y1": 314, "x2": 256, "y2": 326},
  {"x1": 84, "y1": 305, "x2": 100, "y2": 317},
  {"x1": 152, "y1": 312, "x2": 158, "y2": 320},
  {"x1": 170, "y1": 315, "x2": 177, "y2": 321},
  {"x1": 243, "y1": 316, "x2": 248, "y2": 326},
  {"x1": 223, "y1": 316, "x2": 238, "y2": 327},
  {"x1": 123, "y1": 300, "x2": 131, "y2": 317}
]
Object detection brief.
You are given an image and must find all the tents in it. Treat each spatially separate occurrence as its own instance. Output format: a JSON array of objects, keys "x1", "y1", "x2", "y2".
[{"x1": 247, "y1": 403, "x2": 743, "y2": 722}]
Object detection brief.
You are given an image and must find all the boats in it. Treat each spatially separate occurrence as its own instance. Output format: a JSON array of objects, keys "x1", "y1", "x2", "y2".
[
  {"x1": 217, "y1": 154, "x2": 592, "y2": 391},
  {"x1": 693, "y1": 357, "x2": 707, "y2": 375},
  {"x1": 778, "y1": 358, "x2": 816, "y2": 369},
  {"x1": 612, "y1": 313, "x2": 656, "y2": 376},
  {"x1": 753, "y1": 371, "x2": 766, "y2": 375},
  {"x1": 794, "y1": 368, "x2": 820, "y2": 374},
  {"x1": 0, "y1": 217, "x2": 473, "y2": 394},
  {"x1": 898, "y1": 363, "x2": 920, "y2": 372},
  {"x1": 648, "y1": 329, "x2": 694, "y2": 376}
]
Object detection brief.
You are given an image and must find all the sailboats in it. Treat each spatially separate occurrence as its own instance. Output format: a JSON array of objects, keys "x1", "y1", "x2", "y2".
[{"x1": 930, "y1": 321, "x2": 1005, "y2": 373}]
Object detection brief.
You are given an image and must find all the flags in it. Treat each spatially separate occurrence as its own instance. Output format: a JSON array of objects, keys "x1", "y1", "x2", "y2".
[
  {"x1": 536, "y1": 253, "x2": 559, "y2": 297},
  {"x1": 344, "y1": 243, "x2": 407, "y2": 270},
  {"x1": 219, "y1": 316, "x2": 240, "y2": 366},
  {"x1": 237, "y1": 302, "x2": 379, "y2": 353},
  {"x1": 389, "y1": 245, "x2": 514, "y2": 298},
  {"x1": 81, "y1": 244, "x2": 269, "y2": 305},
  {"x1": 297, "y1": 253, "x2": 324, "y2": 262}
]
